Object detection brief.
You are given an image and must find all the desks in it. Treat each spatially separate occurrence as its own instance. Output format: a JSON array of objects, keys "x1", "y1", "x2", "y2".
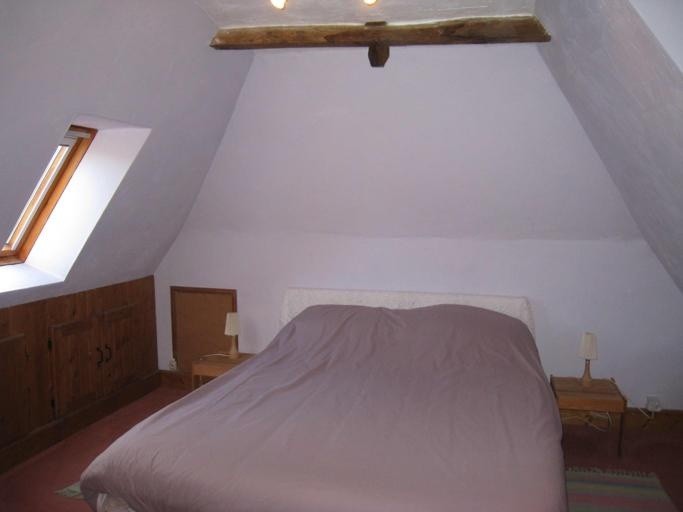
[
  {"x1": 191, "y1": 350, "x2": 256, "y2": 390},
  {"x1": 550, "y1": 378, "x2": 626, "y2": 455}
]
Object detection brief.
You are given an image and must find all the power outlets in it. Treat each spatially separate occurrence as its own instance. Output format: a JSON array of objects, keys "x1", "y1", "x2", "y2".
[{"x1": 169, "y1": 358, "x2": 177, "y2": 369}]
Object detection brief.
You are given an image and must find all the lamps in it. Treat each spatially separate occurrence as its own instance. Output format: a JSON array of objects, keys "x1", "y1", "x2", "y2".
[
  {"x1": 224, "y1": 312, "x2": 241, "y2": 359},
  {"x1": 579, "y1": 332, "x2": 598, "y2": 388}
]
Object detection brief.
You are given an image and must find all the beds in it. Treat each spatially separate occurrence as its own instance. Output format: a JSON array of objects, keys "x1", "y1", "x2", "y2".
[{"x1": 80, "y1": 288, "x2": 567, "y2": 512}]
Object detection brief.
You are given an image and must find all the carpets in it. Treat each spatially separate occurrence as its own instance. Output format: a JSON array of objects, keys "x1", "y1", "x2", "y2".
[{"x1": 53, "y1": 466, "x2": 680, "y2": 512}]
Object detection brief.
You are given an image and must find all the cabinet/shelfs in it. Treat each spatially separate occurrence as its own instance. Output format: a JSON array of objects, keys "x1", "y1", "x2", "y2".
[{"x1": 0, "y1": 275, "x2": 160, "y2": 474}]
